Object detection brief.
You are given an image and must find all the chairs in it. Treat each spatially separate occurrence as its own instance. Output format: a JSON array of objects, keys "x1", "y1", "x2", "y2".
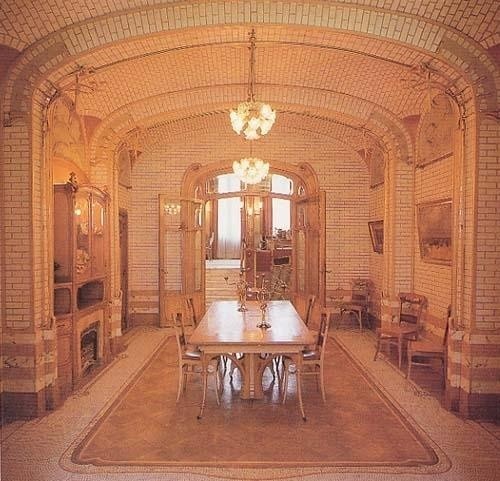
[
  {"x1": 171, "y1": 292, "x2": 333, "y2": 422},
  {"x1": 338, "y1": 278, "x2": 452, "y2": 387}
]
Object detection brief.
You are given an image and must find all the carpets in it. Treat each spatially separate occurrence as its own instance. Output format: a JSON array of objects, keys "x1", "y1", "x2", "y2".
[{"x1": 70, "y1": 335, "x2": 432, "y2": 466}]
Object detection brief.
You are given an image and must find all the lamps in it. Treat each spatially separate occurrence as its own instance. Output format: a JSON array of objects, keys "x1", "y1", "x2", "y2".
[{"x1": 230, "y1": 27, "x2": 276, "y2": 184}]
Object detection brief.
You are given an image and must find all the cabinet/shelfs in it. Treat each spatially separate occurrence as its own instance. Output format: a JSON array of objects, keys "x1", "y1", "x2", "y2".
[{"x1": 53, "y1": 174, "x2": 112, "y2": 398}]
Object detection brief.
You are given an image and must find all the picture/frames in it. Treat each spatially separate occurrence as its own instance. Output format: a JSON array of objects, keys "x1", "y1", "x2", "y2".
[
  {"x1": 413, "y1": 199, "x2": 460, "y2": 267},
  {"x1": 367, "y1": 220, "x2": 386, "y2": 254}
]
